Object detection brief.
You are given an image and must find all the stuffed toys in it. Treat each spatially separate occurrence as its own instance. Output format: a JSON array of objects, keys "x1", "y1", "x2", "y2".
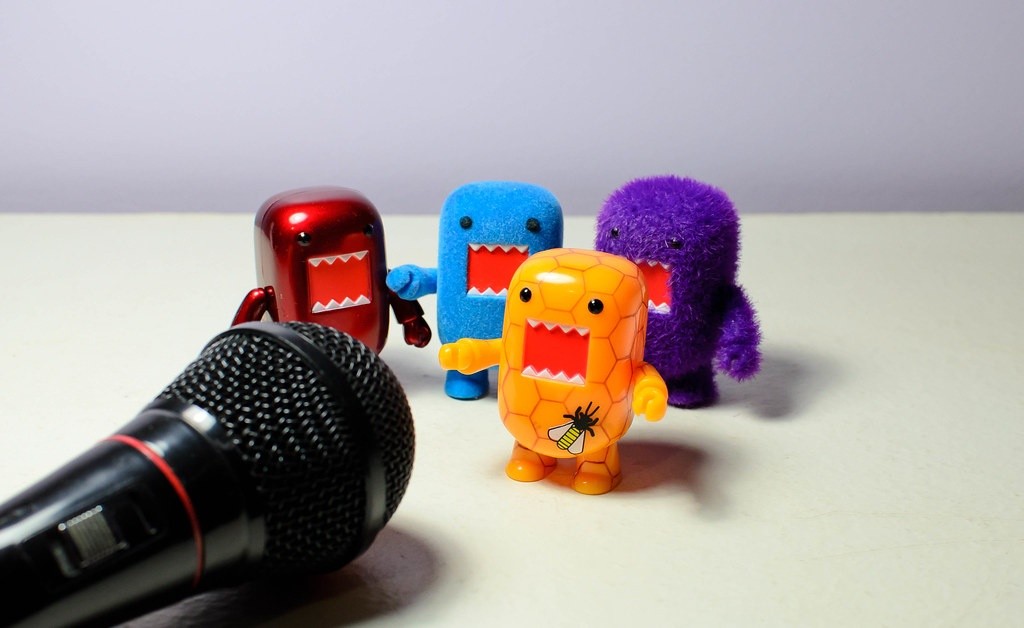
[
  {"x1": 386, "y1": 180, "x2": 563, "y2": 399},
  {"x1": 594, "y1": 176, "x2": 761, "y2": 408}
]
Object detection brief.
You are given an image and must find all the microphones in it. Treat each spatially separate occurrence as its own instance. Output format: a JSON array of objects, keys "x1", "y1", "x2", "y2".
[{"x1": 0, "y1": 320, "x2": 415, "y2": 627}]
